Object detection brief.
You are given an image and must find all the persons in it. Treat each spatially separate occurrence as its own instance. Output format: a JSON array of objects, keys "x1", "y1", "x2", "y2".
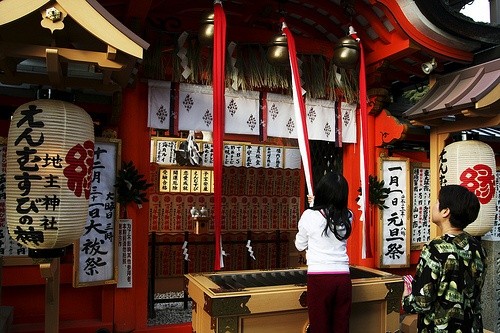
[
  {"x1": 295, "y1": 171, "x2": 354, "y2": 333},
  {"x1": 402, "y1": 185, "x2": 488, "y2": 333}
]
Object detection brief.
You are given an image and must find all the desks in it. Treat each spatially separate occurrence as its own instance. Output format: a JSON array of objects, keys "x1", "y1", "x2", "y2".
[{"x1": 183, "y1": 266, "x2": 406, "y2": 333}]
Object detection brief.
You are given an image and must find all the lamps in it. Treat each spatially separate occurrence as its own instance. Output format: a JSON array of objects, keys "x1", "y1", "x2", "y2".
[{"x1": 421, "y1": 57, "x2": 437, "y2": 74}]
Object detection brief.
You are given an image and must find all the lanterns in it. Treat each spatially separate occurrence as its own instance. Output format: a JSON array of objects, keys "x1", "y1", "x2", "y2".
[
  {"x1": 6, "y1": 88, "x2": 95, "y2": 258},
  {"x1": 439, "y1": 140, "x2": 496, "y2": 242}
]
